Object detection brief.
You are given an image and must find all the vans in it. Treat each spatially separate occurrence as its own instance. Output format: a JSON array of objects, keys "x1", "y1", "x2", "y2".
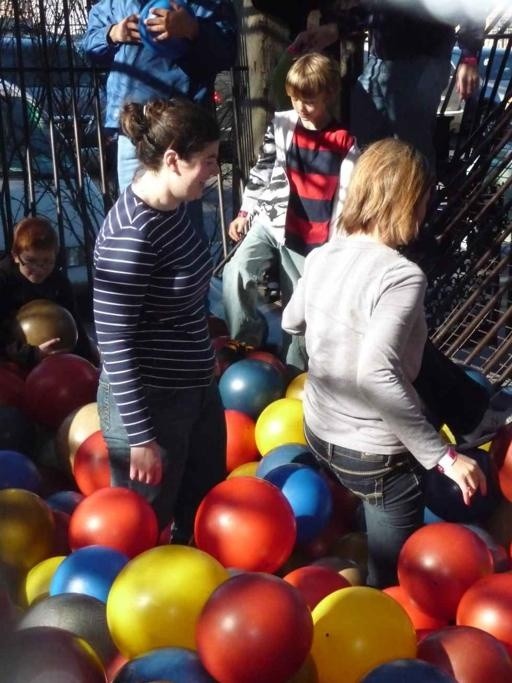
[{"x1": 0, "y1": 32, "x2": 112, "y2": 169}]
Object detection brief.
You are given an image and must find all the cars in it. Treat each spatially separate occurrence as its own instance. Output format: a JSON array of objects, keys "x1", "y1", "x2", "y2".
[
  {"x1": 442, "y1": 49, "x2": 511, "y2": 111},
  {"x1": 1, "y1": 80, "x2": 106, "y2": 309}
]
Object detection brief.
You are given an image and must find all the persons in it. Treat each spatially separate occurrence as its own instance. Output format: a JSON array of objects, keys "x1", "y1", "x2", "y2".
[
  {"x1": 86, "y1": 95, "x2": 230, "y2": 551},
  {"x1": 220, "y1": 55, "x2": 369, "y2": 360},
  {"x1": 288, "y1": 0, "x2": 488, "y2": 168},
  {"x1": 280, "y1": 122, "x2": 512, "y2": 591},
  {"x1": 76, "y1": 0, "x2": 234, "y2": 213},
  {"x1": 0, "y1": 215, "x2": 78, "y2": 354}
]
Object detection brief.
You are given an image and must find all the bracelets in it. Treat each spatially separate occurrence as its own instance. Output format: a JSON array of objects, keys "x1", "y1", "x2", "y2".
[
  {"x1": 461, "y1": 56, "x2": 480, "y2": 64},
  {"x1": 234, "y1": 208, "x2": 250, "y2": 220},
  {"x1": 435, "y1": 448, "x2": 460, "y2": 476}
]
